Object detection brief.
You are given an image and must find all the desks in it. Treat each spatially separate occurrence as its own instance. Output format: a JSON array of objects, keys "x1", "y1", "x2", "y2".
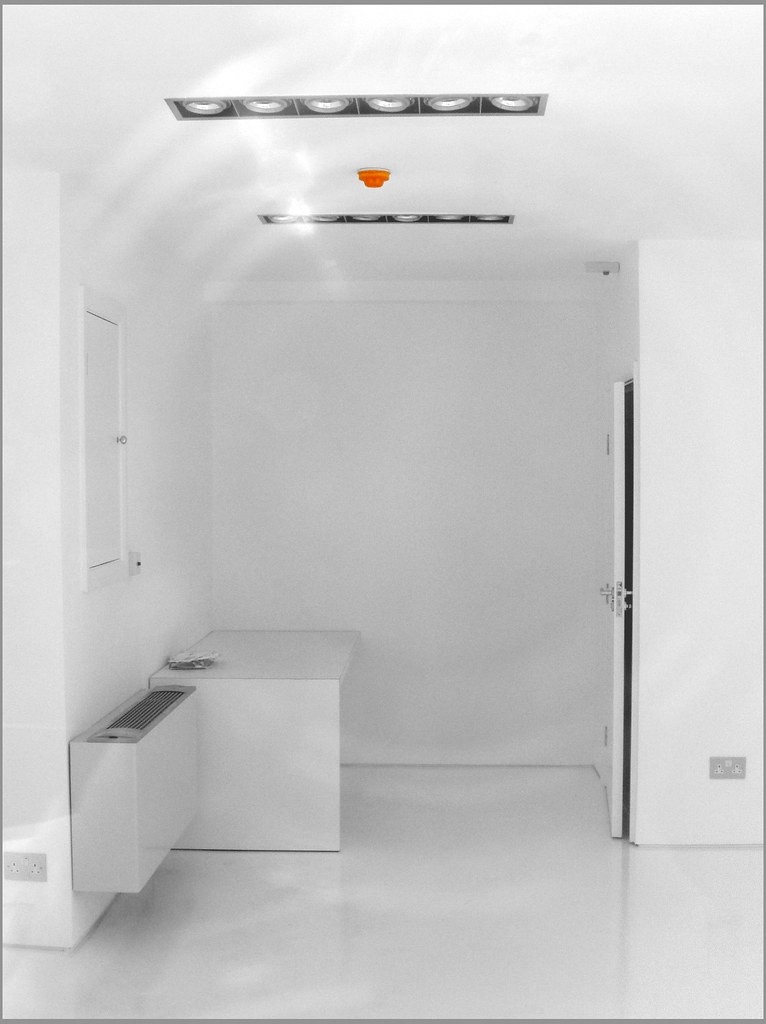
[{"x1": 148, "y1": 631, "x2": 360, "y2": 851}]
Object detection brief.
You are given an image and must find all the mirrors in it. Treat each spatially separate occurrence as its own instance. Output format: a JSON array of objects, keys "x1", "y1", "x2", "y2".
[{"x1": 79, "y1": 286, "x2": 128, "y2": 593}]
